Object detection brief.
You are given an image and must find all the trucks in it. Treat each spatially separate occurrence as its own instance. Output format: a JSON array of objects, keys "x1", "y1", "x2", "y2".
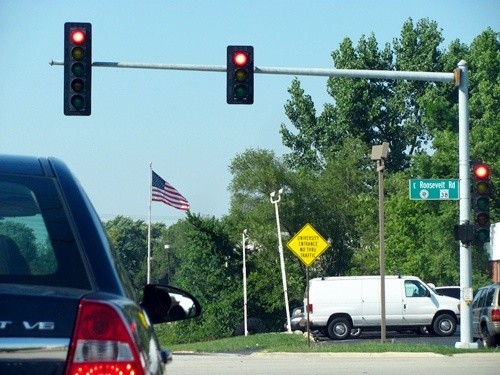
[{"x1": 290, "y1": 272, "x2": 460, "y2": 340}]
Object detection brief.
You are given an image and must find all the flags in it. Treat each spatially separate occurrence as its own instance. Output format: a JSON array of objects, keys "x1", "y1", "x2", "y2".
[{"x1": 149, "y1": 169, "x2": 190, "y2": 210}]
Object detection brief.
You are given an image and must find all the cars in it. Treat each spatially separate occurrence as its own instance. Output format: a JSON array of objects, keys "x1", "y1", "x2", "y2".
[
  {"x1": 472, "y1": 284, "x2": 500, "y2": 348},
  {"x1": 0, "y1": 154, "x2": 202, "y2": 374}
]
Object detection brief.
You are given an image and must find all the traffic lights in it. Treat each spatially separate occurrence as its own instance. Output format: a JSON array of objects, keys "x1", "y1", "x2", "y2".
[
  {"x1": 63, "y1": 22, "x2": 92, "y2": 116},
  {"x1": 227, "y1": 45, "x2": 254, "y2": 104},
  {"x1": 452, "y1": 220, "x2": 473, "y2": 249},
  {"x1": 471, "y1": 162, "x2": 492, "y2": 246}
]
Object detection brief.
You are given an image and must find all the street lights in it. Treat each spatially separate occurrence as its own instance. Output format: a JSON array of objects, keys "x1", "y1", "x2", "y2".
[
  {"x1": 242, "y1": 227, "x2": 250, "y2": 337},
  {"x1": 164, "y1": 244, "x2": 173, "y2": 287},
  {"x1": 269, "y1": 188, "x2": 294, "y2": 333}
]
